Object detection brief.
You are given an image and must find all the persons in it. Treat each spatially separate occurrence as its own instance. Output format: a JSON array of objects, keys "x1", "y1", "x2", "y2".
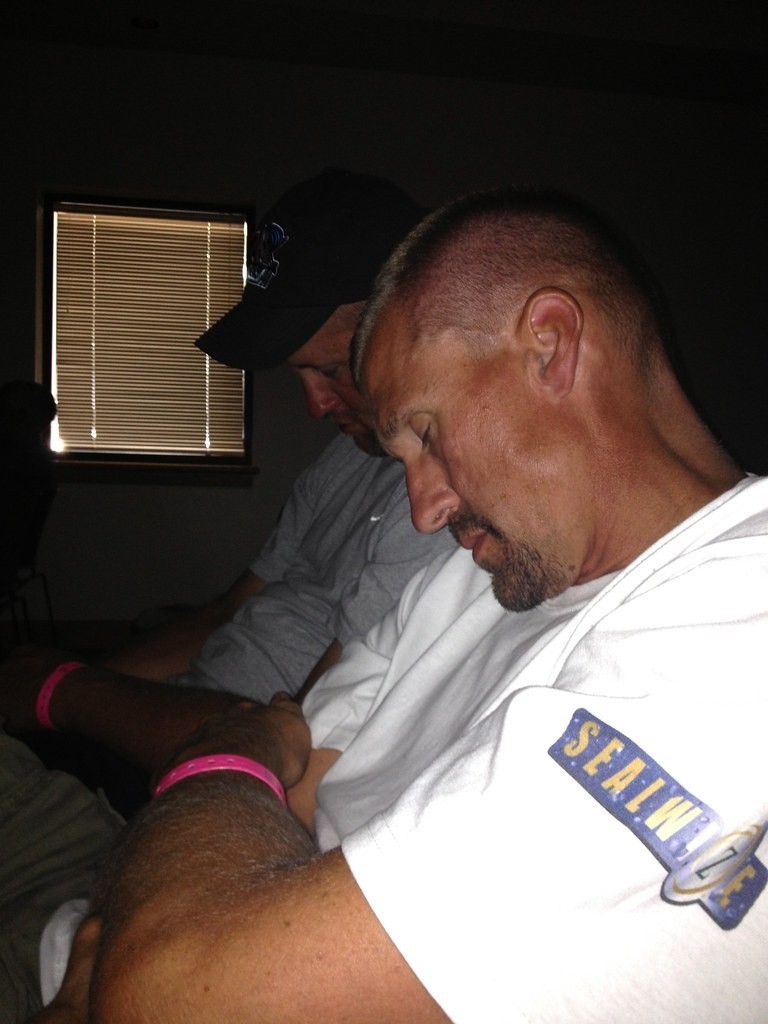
[
  {"x1": 0, "y1": 167, "x2": 433, "y2": 821},
  {"x1": 0, "y1": 183, "x2": 768, "y2": 1023},
  {"x1": 0, "y1": 379, "x2": 56, "y2": 591}
]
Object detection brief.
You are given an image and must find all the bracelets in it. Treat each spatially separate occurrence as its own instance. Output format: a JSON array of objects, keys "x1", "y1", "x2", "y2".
[
  {"x1": 35, "y1": 658, "x2": 90, "y2": 731},
  {"x1": 153, "y1": 754, "x2": 286, "y2": 809}
]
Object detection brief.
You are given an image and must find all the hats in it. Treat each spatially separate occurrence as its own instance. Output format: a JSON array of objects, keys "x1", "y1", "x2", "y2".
[{"x1": 195, "y1": 168, "x2": 433, "y2": 372}]
